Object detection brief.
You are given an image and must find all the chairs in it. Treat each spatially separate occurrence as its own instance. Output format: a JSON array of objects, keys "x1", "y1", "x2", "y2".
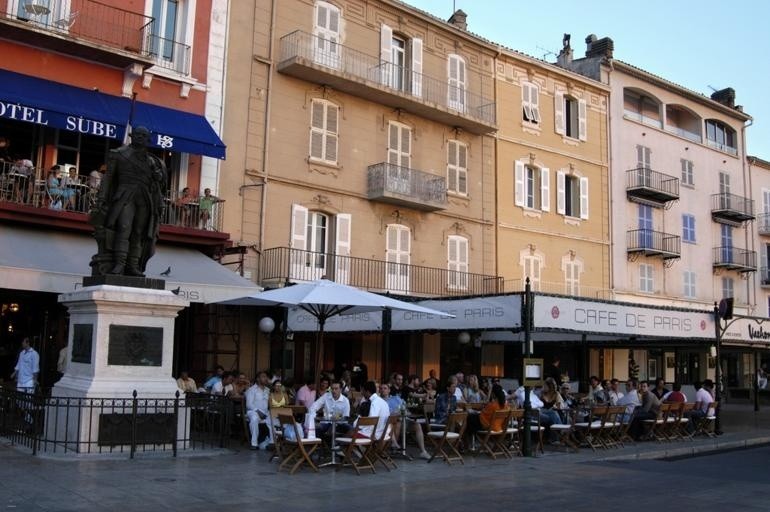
[
  {"x1": 187, "y1": 390, "x2": 269, "y2": 450},
  {"x1": 55, "y1": 10, "x2": 80, "y2": 31},
  {"x1": 548, "y1": 394, "x2": 719, "y2": 453},
  {"x1": 269, "y1": 391, "x2": 406, "y2": 474},
  {"x1": 1, "y1": 164, "x2": 207, "y2": 232},
  {"x1": 406, "y1": 394, "x2": 546, "y2": 467}
]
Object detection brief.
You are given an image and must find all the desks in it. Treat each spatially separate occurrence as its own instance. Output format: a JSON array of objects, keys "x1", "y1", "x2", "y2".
[{"x1": 23, "y1": 4, "x2": 50, "y2": 23}]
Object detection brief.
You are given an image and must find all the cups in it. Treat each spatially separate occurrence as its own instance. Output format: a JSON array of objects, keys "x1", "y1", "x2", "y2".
[
  {"x1": 401, "y1": 396, "x2": 439, "y2": 407},
  {"x1": 556, "y1": 398, "x2": 598, "y2": 409},
  {"x1": 325, "y1": 405, "x2": 344, "y2": 420},
  {"x1": 505, "y1": 399, "x2": 525, "y2": 409},
  {"x1": 453, "y1": 395, "x2": 491, "y2": 413}
]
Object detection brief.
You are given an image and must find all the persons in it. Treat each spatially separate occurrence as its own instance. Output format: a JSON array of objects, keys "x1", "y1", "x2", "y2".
[
  {"x1": 89, "y1": 164, "x2": 102, "y2": 210},
  {"x1": 174, "y1": 187, "x2": 197, "y2": 228},
  {"x1": 757, "y1": 363, "x2": 768, "y2": 389},
  {"x1": 96, "y1": 124, "x2": 171, "y2": 277},
  {"x1": 47, "y1": 165, "x2": 65, "y2": 211},
  {"x1": 198, "y1": 188, "x2": 220, "y2": 231},
  {"x1": 8, "y1": 154, "x2": 34, "y2": 203},
  {"x1": 56, "y1": 347, "x2": 68, "y2": 376},
  {"x1": 60, "y1": 167, "x2": 83, "y2": 212},
  {"x1": 10, "y1": 337, "x2": 40, "y2": 434}
]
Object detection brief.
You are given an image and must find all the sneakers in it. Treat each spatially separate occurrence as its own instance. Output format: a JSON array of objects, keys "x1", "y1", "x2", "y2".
[
  {"x1": 419, "y1": 451, "x2": 432, "y2": 460},
  {"x1": 250, "y1": 446, "x2": 257, "y2": 451}
]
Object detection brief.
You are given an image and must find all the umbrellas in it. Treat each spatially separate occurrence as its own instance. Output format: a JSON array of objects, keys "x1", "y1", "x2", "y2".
[{"x1": 204, "y1": 275, "x2": 456, "y2": 400}]
